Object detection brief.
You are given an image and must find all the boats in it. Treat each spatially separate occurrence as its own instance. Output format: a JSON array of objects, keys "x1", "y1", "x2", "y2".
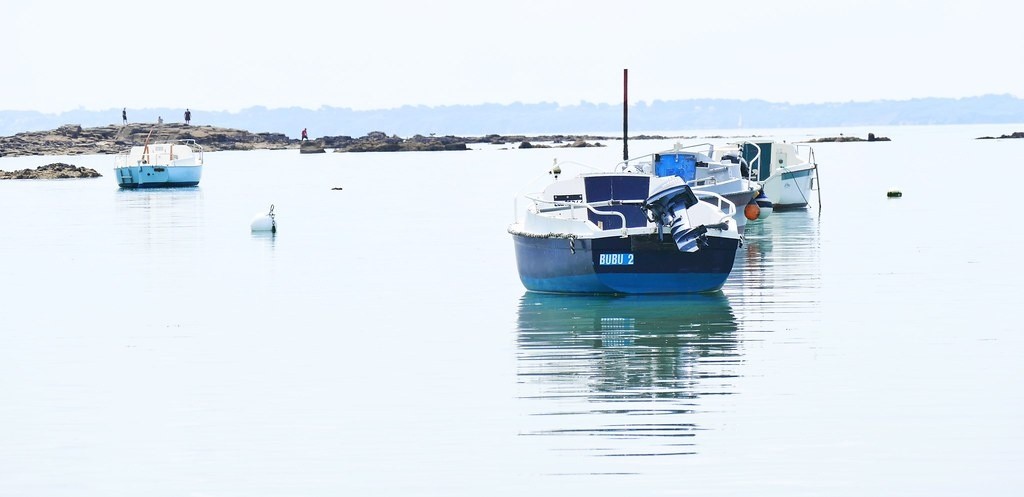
[
  {"x1": 616, "y1": 141, "x2": 763, "y2": 228},
  {"x1": 113, "y1": 123, "x2": 204, "y2": 188},
  {"x1": 698, "y1": 141, "x2": 818, "y2": 212}
]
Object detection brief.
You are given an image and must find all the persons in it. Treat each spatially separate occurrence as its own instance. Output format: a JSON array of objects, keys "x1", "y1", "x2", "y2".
[
  {"x1": 158, "y1": 116, "x2": 163, "y2": 124},
  {"x1": 123, "y1": 107, "x2": 128, "y2": 124},
  {"x1": 184, "y1": 109, "x2": 191, "y2": 123},
  {"x1": 302, "y1": 128, "x2": 308, "y2": 140}
]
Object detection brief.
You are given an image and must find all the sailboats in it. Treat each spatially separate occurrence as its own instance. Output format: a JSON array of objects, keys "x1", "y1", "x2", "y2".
[{"x1": 506, "y1": 69, "x2": 741, "y2": 296}]
